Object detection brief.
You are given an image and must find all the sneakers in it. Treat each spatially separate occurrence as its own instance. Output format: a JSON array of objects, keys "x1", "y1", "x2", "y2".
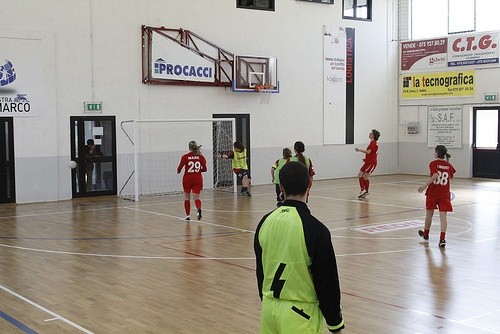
[
  {"x1": 197, "y1": 208, "x2": 202, "y2": 221},
  {"x1": 239, "y1": 190, "x2": 251, "y2": 196},
  {"x1": 419, "y1": 230, "x2": 429, "y2": 240},
  {"x1": 358, "y1": 190, "x2": 368, "y2": 198},
  {"x1": 185, "y1": 215, "x2": 190, "y2": 220},
  {"x1": 439, "y1": 240, "x2": 447, "y2": 247}
]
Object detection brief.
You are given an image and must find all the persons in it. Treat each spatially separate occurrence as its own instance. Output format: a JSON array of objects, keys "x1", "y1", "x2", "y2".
[
  {"x1": 355, "y1": 129, "x2": 380, "y2": 198},
  {"x1": 217, "y1": 141, "x2": 252, "y2": 197},
  {"x1": 418, "y1": 145, "x2": 456, "y2": 246},
  {"x1": 254, "y1": 141, "x2": 345, "y2": 334},
  {"x1": 177, "y1": 141, "x2": 207, "y2": 221},
  {"x1": 84, "y1": 140, "x2": 104, "y2": 191}
]
